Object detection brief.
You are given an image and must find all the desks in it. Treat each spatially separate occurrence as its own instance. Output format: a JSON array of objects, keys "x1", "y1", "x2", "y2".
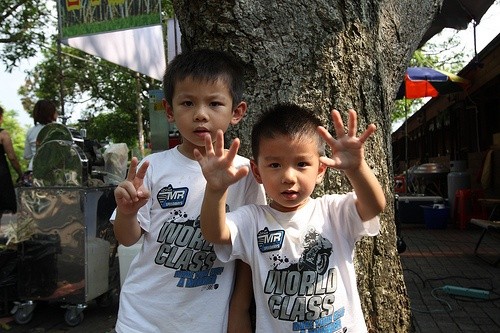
[{"x1": 393, "y1": 193, "x2": 443, "y2": 253}]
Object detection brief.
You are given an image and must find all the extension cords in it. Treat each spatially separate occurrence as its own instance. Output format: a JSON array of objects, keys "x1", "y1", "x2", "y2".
[{"x1": 443, "y1": 285, "x2": 490, "y2": 298}]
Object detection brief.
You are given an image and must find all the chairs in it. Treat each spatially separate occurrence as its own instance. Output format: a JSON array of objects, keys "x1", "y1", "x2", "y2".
[{"x1": 470, "y1": 198, "x2": 500, "y2": 254}]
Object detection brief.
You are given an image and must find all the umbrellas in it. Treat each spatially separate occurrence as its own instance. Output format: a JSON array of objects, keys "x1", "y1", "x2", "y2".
[{"x1": 393, "y1": 66, "x2": 469, "y2": 162}]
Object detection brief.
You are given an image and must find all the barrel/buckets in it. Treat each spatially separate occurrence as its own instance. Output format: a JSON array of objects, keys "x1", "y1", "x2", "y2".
[{"x1": 424, "y1": 204, "x2": 452, "y2": 230}]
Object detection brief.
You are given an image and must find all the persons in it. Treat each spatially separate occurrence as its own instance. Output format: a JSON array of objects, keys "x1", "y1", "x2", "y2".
[
  {"x1": 98, "y1": 47, "x2": 269, "y2": 333},
  {"x1": 22, "y1": 98, "x2": 59, "y2": 176},
  {"x1": 193, "y1": 101, "x2": 388, "y2": 333},
  {"x1": 0, "y1": 107, "x2": 26, "y2": 216}
]
